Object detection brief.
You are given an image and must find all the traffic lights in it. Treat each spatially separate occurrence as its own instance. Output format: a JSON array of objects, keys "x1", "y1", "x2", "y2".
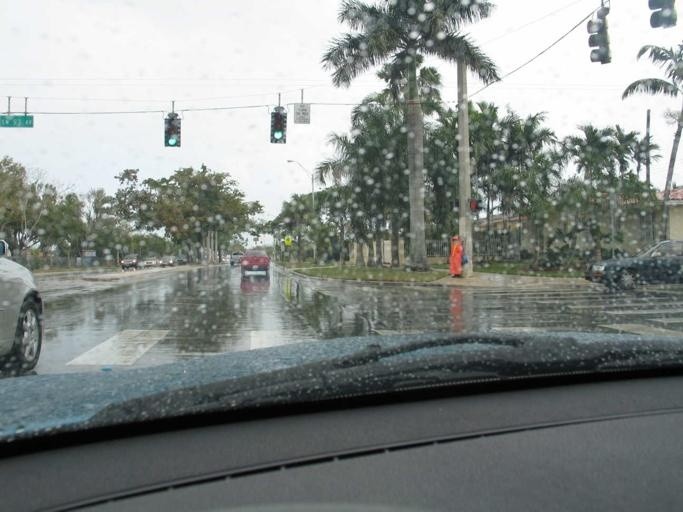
[
  {"x1": 469, "y1": 199, "x2": 483, "y2": 212},
  {"x1": 163, "y1": 118, "x2": 180, "y2": 148},
  {"x1": 586, "y1": 4, "x2": 611, "y2": 66},
  {"x1": 647, "y1": 0, "x2": 675, "y2": 29},
  {"x1": 269, "y1": 112, "x2": 287, "y2": 144}
]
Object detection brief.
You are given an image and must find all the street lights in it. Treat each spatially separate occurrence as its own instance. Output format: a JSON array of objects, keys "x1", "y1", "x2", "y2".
[{"x1": 285, "y1": 156, "x2": 318, "y2": 266}]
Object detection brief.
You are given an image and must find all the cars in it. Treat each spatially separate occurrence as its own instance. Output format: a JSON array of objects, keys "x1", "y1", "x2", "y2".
[
  {"x1": 584, "y1": 238, "x2": 682, "y2": 293},
  {"x1": 241, "y1": 249, "x2": 271, "y2": 277},
  {"x1": 0, "y1": 240, "x2": 44, "y2": 369},
  {"x1": 1, "y1": 323, "x2": 681, "y2": 511},
  {"x1": 120, "y1": 250, "x2": 190, "y2": 270},
  {"x1": 239, "y1": 276, "x2": 272, "y2": 297}
]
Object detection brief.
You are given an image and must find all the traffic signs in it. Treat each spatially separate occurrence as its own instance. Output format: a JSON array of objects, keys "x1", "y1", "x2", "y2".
[
  {"x1": 0, "y1": 114, "x2": 34, "y2": 128},
  {"x1": 293, "y1": 102, "x2": 309, "y2": 125}
]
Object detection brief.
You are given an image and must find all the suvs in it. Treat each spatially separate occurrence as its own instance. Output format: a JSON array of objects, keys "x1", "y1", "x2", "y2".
[{"x1": 229, "y1": 250, "x2": 243, "y2": 266}]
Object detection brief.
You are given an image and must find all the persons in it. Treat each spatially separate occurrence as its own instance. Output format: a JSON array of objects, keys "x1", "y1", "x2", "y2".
[{"x1": 449, "y1": 235, "x2": 463, "y2": 278}]
